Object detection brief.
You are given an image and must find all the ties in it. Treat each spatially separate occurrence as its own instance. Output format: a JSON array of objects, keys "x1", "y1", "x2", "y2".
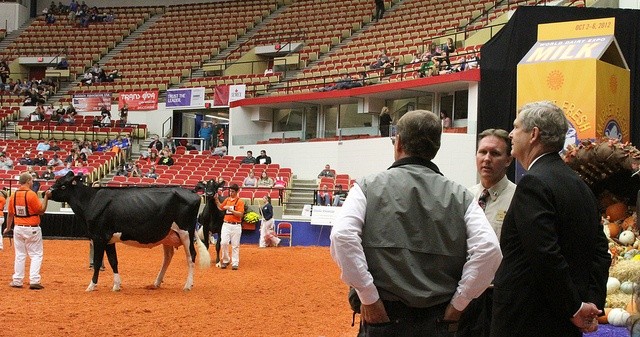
[{"x1": 478, "y1": 189, "x2": 490, "y2": 211}]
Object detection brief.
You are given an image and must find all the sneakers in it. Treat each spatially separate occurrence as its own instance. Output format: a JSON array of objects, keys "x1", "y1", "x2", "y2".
[
  {"x1": 232, "y1": 265, "x2": 237, "y2": 269},
  {"x1": 221, "y1": 263, "x2": 229, "y2": 268},
  {"x1": 9, "y1": 281, "x2": 22, "y2": 287},
  {"x1": 29, "y1": 283, "x2": 43, "y2": 289}
]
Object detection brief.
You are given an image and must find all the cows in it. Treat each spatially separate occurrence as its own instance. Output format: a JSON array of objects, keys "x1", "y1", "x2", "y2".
[
  {"x1": 42, "y1": 171, "x2": 210, "y2": 292},
  {"x1": 195, "y1": 181, "x2": 226, "y2": 263}
]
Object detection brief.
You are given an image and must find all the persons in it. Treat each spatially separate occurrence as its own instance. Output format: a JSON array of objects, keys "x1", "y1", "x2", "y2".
[
  {"x1": 379, "y1": 105, "x2": 392, "y2": 136},
  {"x1": 441, "y1": 110, "x2": 452, "y2": 129},
  {"x1": 259, "y1": 195, "x2": 278, "y2": 248},
  {"x1": 490, "y1": 102, "x2": 611, "y2": 335},
  {"x1": 3, "y1": 172, "x2": 57, "y2": 288},
  {"x1": 318, "y1": 164, "x2": 335, "y2": 177},
  {"x1": 330, "y1": 109, "x2": 504, "y2": 337},
  {"x1": 0, "y1": 188, "x2": 7, "y2": 229},
  {"x1": 466, "y1": 129, "x2": 516, "y2": 237},
  {"x1": 214, "y1": 184, "x2": 244, "y2": 271},
  {"x1": 316, "y1": 183, "x2": 330, "y2": 206},
  {"x1": 380, "y1": 35, "x2": 479, "y2": 77},
  {"x1": 333, "y1": 183, "x2": 347, "y2": 206}
]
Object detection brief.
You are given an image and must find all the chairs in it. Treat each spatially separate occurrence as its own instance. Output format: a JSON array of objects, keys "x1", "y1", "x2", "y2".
[
  {"x1": 14, "y1": 111, "x2": 147, "y2": 141},
  {"x1": 0, "y1": 139, "x2": 131, "y2": 192},
  {"x1": 180, "y1": 1, "x2": 397, "y2": 101},
  {"x1": 0, "y1": 5, "x2": 166, "y2": 84},
  {"x1": 466, "y1": 0, "x2": 597, "y2": 36},
  {"x1": 103, "y1": 145, "x2": 293, "y2": 219},
  {"x1": 275, "y1": 222, "x2": 293, "y2": 247},
  {"x1": 0, "y1": 82, "x2": 57, "y2": 131},
  {"x1": 270, "y1": 0, "x2": 481, "y2": 96},
  {"x1": 53, "y1": 0, "x2": 278, "y2": 107},
  {"x1": 314, "y1": 167, "x2": 357, "y2": 207}
]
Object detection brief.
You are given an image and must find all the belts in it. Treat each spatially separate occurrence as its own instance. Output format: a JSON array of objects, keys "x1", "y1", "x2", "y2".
[
  {"x1": 224, "y1": 220, "x2": 243, "y2": 225},
  {"x1": 18, "y1": 224, "x2": 38, "y2": 227}
]
